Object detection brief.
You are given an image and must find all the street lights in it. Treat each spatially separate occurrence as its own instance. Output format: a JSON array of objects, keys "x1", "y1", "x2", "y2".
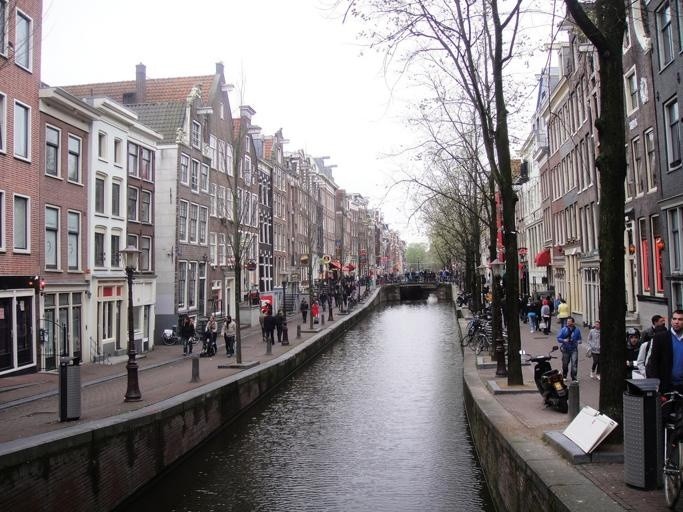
[
  {"x1": 118, "y1": 245, "x2": 142, "y2": 401},
  {"x1": 478, "y1": 263, "x2": 488, "y2": 316},
  {"x1": 490, "y1": 259, "x2": 508, "y2": 378},
  {"x1": 280, "y1": 272, "x2": 289, "y2": 345}
]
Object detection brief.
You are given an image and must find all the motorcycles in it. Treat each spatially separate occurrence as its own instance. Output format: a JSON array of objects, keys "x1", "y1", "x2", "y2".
[
  {"x1": 456, "y1": 290, "x2": 473, "y2": 310},
  {"x1": 518, "y1": 346, "x2": 568, "y2": 413}
]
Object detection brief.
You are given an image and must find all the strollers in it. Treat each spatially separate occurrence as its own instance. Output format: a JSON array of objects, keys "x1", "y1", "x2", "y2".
[{"x1": 200, "y1": 330, "x2": 216, "y2": 358}]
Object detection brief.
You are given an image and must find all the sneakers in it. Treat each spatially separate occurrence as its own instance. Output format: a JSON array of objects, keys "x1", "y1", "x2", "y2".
[
  {"x1": 591, "y1": 371, "x2": 600, "y2": 381},
  {"x1": 563, "y1": 377, "x2": 579, "y2": 384}
]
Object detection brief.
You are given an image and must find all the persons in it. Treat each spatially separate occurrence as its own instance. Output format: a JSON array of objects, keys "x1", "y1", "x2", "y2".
[
  {"x1": 638, "y1": 314, "x2": 666, "y2": 340},
  {"x1": 586, "y1": 319, "x2": 600, "y2": 380},
  {"x1": 457, "y1": 268, "x2": 570, "y2": 336},
  {"x1": 274, "y1": 309, "x2": 286, "y2": 342},
  {"x1": 369, "y1": 268, "x2": 456, "y2": 287},
  {"x1": 624, "y1": 327, "x2": 642, "y2": 382},
  {"x1": 180, "y1": 316, "x2": 195, "y2": 357},
  {"x1": 219, "y1": 315, "x2": 236, "y2": 358},
  {"x1": 555, "y1": 316, "x2": 583, "y2": 384},
  {"x1": 649, "y1": 308, "x2": 683, "y2": 443},
  {"x1": 636, "y1": 326, "x2": 668, "y2": 378},
  {"x1": 258, "y1": 310, "x2": 269, "y2": 342},
  {"x1": 263, "y1": 310, "x2": 276, "y2": 346},
  {"x1": 300, "y1": 271, "x2": 369, "y2": 323},
  {"x1": 204, "y1": 314, "x2": 218, "y2": 354}
]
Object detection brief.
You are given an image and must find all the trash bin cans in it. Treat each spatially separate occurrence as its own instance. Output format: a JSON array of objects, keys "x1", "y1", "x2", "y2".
[
  {"x1": 623, "y1": 378, "x2": 665, "y2": 490},
  {"x1": 58, "y1": 357, "x2": 81, "y2": 422}
]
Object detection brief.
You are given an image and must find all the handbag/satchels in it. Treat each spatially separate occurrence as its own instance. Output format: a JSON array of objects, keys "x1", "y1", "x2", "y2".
[
  {"x1": 539, "y1": 322, "x2": 547, "y2": 329},
  {"x1": 586, "y1": 347, "x2": 593, "y2": 358}
]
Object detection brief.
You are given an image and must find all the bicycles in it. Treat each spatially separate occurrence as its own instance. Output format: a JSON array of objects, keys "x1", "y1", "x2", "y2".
[
  {"x1": 162, "y1": 325, "x2": 200, "y2": 345},
  {"x1": 459, "y1": 307, "x2": 508, "y2": 356},
  {"x1": 661, "y1": 391, "x2": 683, "y2": 506}
]
